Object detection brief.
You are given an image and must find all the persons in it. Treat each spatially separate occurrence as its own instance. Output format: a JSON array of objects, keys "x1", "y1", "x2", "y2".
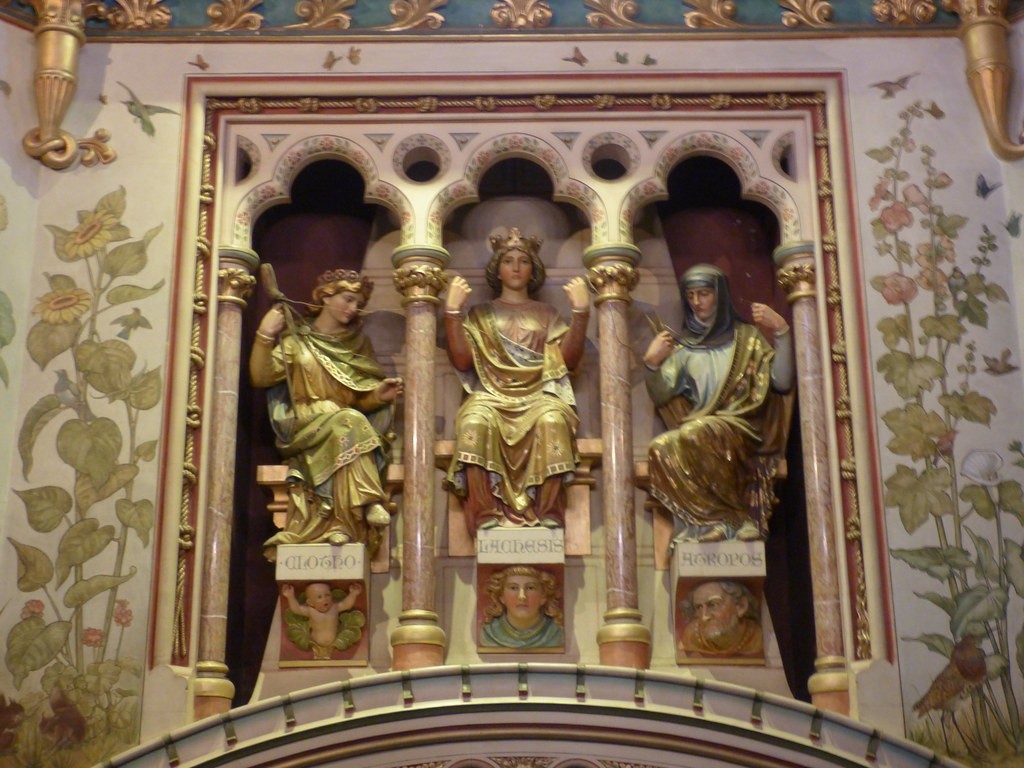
[
  {"x1": 278, "y1": 578, "x2": 365, "y2": 661},
  {"x1": 479, "y1": 567, "x2": 564, "y2": 651},
  {"x1": 443, "y1": 227, "x2": 591, "y2": 531},
  {"x1": 248, "y1": 267, "x2": 406, "y2": 547},
  {"x1": 678, "y1": 579, "x2": 764, "y2": 663},
  {"x1": 641, "y1": 263, "x2": 797, "y2": 542}
]
